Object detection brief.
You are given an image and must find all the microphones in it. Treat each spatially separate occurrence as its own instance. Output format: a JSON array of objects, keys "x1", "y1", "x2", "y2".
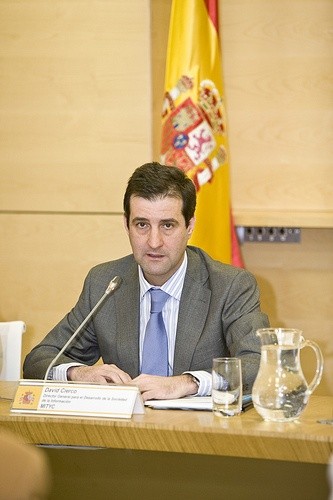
[{"x1": 44, "y1": 275, "x2": 124, "y2": 380}]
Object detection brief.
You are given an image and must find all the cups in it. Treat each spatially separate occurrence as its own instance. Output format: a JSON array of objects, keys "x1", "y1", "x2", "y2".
[{"x1": 212, "y1": 357, "x2": 242, "y2": 417}]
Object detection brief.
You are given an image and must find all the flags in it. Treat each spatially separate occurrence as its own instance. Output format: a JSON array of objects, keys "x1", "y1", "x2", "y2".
[{"x1": 161, "y1": 0, "x2": 245, "y2": 269}]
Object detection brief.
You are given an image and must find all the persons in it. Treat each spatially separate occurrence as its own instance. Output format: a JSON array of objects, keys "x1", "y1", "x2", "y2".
[{"x1": 22, "y1": 162, "x2": 272, "y2": 402}]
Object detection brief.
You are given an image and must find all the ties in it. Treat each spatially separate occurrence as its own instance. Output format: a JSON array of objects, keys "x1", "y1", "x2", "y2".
[{"x1": 139, "y1": 290, "x2": 170, "y2": 378}]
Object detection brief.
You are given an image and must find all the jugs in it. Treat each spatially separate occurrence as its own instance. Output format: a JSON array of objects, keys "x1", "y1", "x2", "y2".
[{"x1": 252, "y1": 327, "x2": 323, "y2": 423}]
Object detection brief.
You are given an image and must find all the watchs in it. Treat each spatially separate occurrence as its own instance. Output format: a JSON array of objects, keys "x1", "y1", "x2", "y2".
[{"x1": 192, "y1": 376, "x2": 200, "y2": 387}]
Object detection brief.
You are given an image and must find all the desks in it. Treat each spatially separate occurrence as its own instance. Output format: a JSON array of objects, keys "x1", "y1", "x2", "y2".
[{"x1": 1, "y1": 394, "x2": 333, "y2": 500}]
